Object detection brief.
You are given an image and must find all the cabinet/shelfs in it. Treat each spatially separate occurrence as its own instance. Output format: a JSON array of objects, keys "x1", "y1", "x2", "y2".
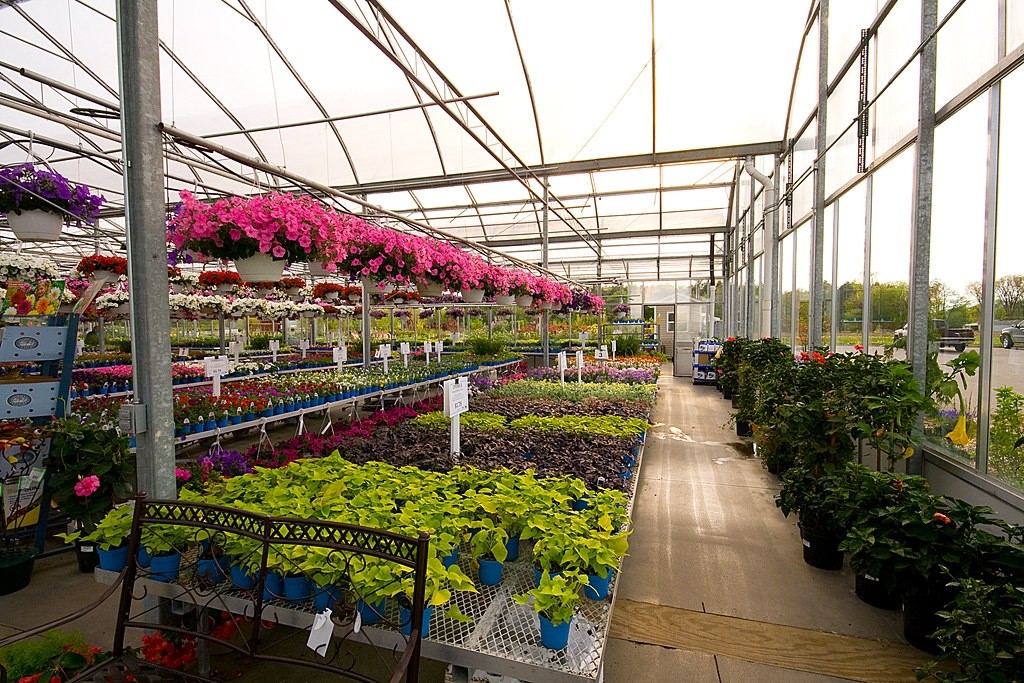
[
  {"x1": 690, "y1": 349, "x2": 718, "y2": 385},
  {"x1": 601, "y1": 323, "x2": 660, "y2": 356}
]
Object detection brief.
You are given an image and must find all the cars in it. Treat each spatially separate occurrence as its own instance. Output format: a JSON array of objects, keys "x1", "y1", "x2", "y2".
[{"x1": 999, "y1": 319, "x2": 1024, "y2": 349}]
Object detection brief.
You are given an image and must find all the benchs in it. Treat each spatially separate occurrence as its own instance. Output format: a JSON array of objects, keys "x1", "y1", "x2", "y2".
[{"x1": 71, "y1": 489, "x2": 432, "y2": 683}]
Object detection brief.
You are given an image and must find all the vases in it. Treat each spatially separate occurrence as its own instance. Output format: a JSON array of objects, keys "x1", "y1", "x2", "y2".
[
  {"x1": 0, "y1": 209, "x2": 647, "y2": 655},
  {"x1": 715, "y1": 383, "x2": 973, "y2": 654}
]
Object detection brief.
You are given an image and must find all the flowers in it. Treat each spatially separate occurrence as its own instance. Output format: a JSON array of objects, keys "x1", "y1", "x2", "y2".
[
  {"x1": 0, "y1": 162, "x2": 668, "y2": 543},
  {"x1": 0, "y1": 603, "x2": 279, "y2": 683},
  {"x1": 714, "y1": 334, "x2": 1005, "y2": 573}
]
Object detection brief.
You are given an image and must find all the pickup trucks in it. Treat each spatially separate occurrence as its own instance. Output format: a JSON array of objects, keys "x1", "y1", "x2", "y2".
[{"x1": 893, "y1": 318, "x2": 976, "y2": 352}]
